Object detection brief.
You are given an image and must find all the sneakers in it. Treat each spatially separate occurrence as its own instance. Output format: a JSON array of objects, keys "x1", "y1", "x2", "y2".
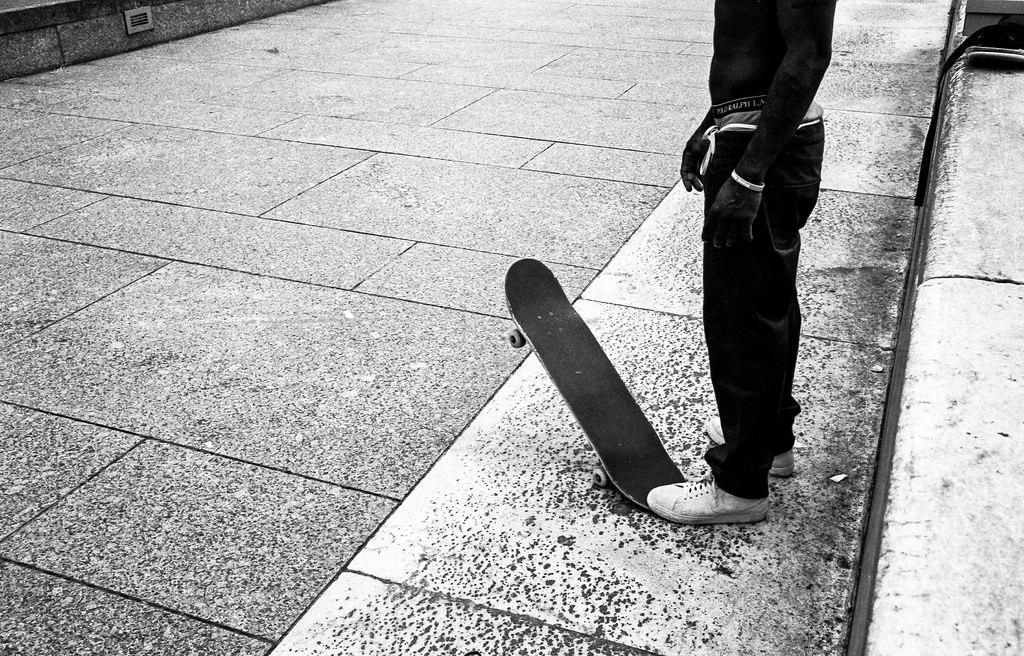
[
  {"x1": 706, "y1": 417, "x2": 794, "y2": 477},
  {"x1": 647, "y1": 469, "x2": 768, "y2": 524}
]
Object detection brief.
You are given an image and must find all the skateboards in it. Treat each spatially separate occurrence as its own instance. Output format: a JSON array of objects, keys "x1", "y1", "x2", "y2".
[{"x1": 504, "y1": 259, "x2": 686, "y2": 512}]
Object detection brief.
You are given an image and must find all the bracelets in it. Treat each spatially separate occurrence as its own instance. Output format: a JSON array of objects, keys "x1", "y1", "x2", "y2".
[{"x1": 730, "y1": 169, "x2": 765, "y2": 191}]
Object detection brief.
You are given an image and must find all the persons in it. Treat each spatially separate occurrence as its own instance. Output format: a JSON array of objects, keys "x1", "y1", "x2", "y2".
[{"x1": 646, "y1": 0, "x2": 839, "y2": 525}]
